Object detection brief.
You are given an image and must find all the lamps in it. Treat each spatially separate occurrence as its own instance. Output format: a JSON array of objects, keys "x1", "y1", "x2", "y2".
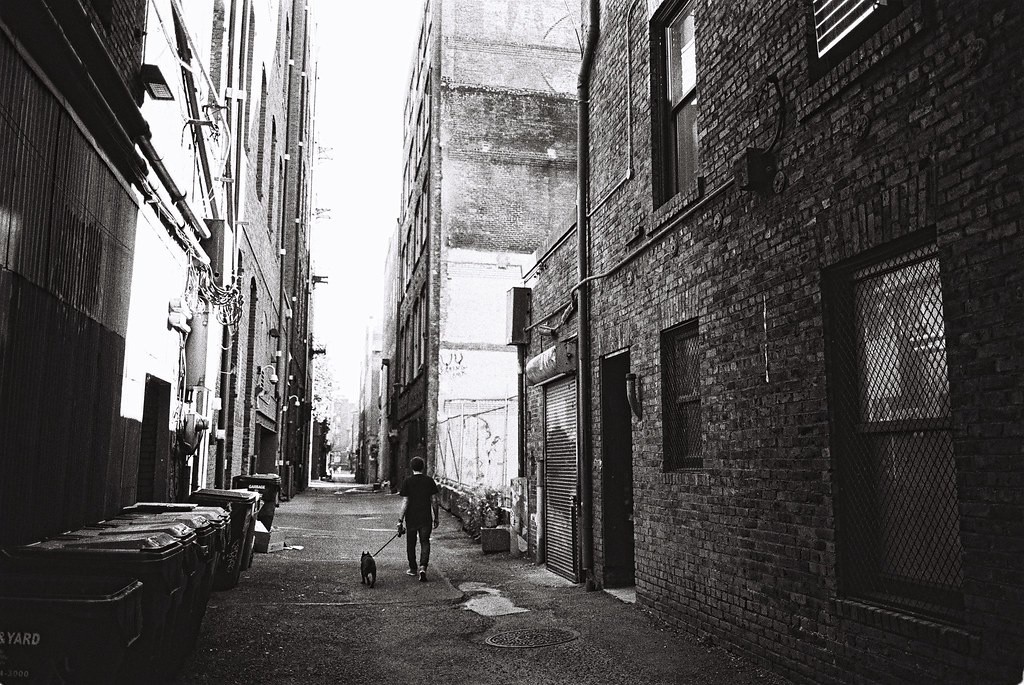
[
  {"x1": 268, "y1": 328, "x2": 281, "y2": 338},
  {"x1": 256, "y1": 363, "x2": 278, "y2": 385},
  {"x1": 289, "y1": 395, "x2": 301, "y2": 407},
  {"x1": 136, "y1": 63, "x2": 175, "y2": 102}
]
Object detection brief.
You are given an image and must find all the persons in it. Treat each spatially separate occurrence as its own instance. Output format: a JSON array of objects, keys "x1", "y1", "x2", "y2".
[{"x1": 397, "y1": 457, "x2": 439, "y2": 581}]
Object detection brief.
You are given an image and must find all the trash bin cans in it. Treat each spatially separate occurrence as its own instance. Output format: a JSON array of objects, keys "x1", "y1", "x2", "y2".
[
  {"x1": 187, "y1": 487, "x2": 263, "y2": 591},
  {"x1": 232, "y1": 473, "x2": 283, "y2": 553},
  {"x1": 0, "y1": 501, "x2": 232, "y2": 685}
]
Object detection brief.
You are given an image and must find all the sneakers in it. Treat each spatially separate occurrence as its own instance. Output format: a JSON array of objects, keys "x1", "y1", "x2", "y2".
[
  {"x1": 406, "y1": 568, "x2": 418, "y2": 576},
  {"x1": 418, "y1": 565, "x2": 428, "y2": 582}
]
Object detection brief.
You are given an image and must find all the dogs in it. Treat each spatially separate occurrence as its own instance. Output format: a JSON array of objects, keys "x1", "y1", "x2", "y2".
[{"x1": 360, "y1": 551, "x2": 377, "y2": 589}]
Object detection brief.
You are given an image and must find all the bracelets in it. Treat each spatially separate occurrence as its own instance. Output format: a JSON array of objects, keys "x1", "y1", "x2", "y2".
[{"x1": 398, "y1": 519, "x2": 402, "y2": 522}]
